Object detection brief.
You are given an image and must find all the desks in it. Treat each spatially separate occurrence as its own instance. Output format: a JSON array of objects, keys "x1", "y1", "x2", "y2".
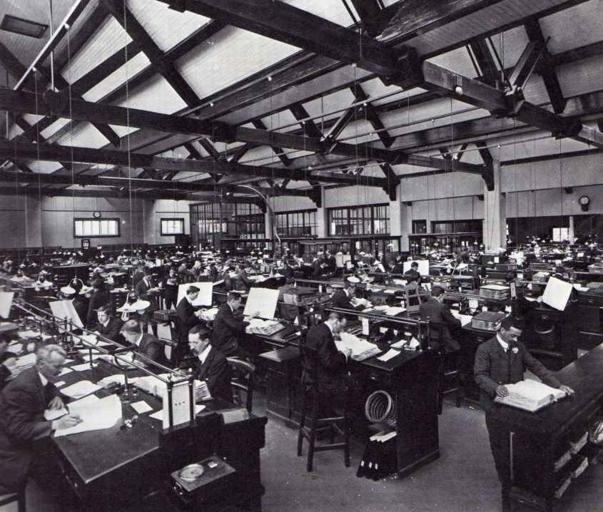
[{"x1": 3, "y1": 232, "x2": 602, "y2": 511}]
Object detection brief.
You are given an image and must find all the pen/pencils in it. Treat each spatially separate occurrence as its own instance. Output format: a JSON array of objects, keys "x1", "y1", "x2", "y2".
[{"x1": 499, "y1": 381, "x2": 503, "y2": 401}]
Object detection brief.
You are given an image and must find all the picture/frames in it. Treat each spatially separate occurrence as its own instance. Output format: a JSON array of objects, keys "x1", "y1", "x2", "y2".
[
  {"x1": 159, "y1": 217, "x2": 185, "y2": 236},
  {"x1": 74, "y1": 217, "x2": 121, "y2": 238}
]
